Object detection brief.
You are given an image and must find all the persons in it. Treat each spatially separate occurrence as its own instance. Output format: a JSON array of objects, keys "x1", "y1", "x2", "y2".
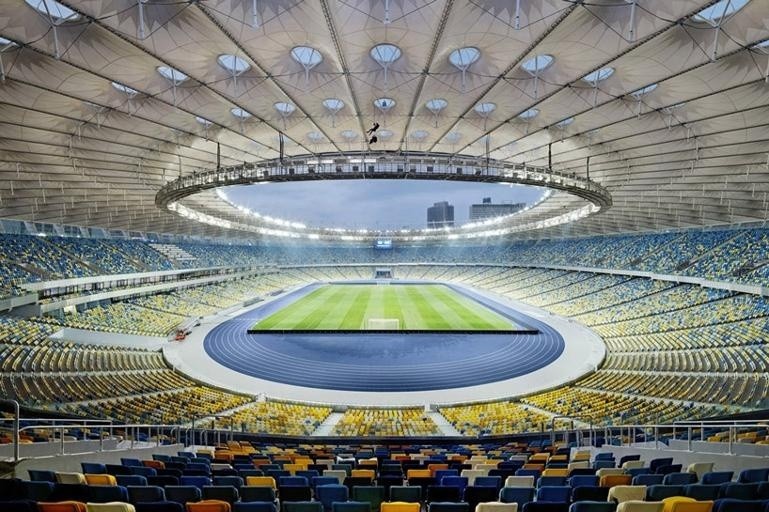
[
  {"x1": 368, "y1": 136, "x2": 378, "y2": 145},
  {"x1": 381, "y1": 99, "x2": 387, "y2": 108},
  {"x1": 365, "y1": 123, "x2": 379, "y2": 136}
]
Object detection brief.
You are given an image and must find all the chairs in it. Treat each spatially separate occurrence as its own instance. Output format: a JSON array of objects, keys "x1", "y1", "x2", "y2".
[{"x1": 0, "y1": 226, "x2": 769, "y2": 512}]
